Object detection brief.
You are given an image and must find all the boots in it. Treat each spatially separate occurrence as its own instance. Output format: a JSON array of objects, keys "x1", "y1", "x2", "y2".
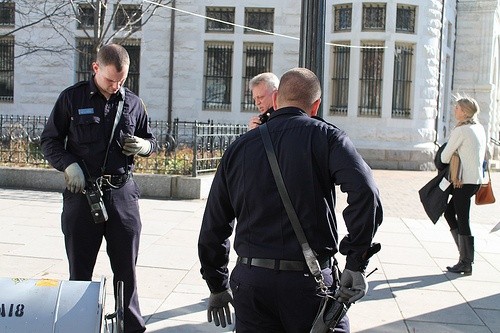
[
  {"x1": 446, "y1": 236, "x2": 474, "y2": 275},
  {"x1": 451, "y1": 226, "x2": 460, "y2": 248}
]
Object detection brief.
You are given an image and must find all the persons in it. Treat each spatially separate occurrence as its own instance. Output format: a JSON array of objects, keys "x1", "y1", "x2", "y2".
[
  {"x1": 198, "y1": 67, "x2": 383, "y2": 333},
  {"x1": 441, "y1": 98, "x2": 486, "y2": 274},
  {"x1": 247, "y1": 73, "x2": 280, "y2": 131},
  {"x1": 39, "y1": 44, "x2": 156, "y2": 333}
]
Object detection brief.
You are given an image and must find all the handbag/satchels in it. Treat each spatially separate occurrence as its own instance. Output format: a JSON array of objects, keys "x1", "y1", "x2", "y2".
[
  {"x1": 474, "y1": 182, "x2": 495, "y2": 204},
  {"x1": 309, "y1": 294, "x2": 349, "y2": 333}
]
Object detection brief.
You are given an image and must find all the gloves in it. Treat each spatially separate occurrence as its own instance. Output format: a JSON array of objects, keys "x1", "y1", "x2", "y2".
[
  {"x1": 207, "y1": 290, "x2": 236, "y2": 328},
  {"x1": 122, "y1": 134, "x2": 151, "y2": 157},
  {"x1": 64, "y1": 162, "x2": 86, "y2": 194},
  {"x1": 336, "y1": 269, "x2": 367, "y2": 303}
]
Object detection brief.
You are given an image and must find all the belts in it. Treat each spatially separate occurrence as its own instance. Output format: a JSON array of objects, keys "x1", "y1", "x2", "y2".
[
  {"x1": 238, "y1": 258, "x2": 330, "y2": 271},
  {"x1": 85, "y1": 172, "x2": 132, "y2": 189}
]
